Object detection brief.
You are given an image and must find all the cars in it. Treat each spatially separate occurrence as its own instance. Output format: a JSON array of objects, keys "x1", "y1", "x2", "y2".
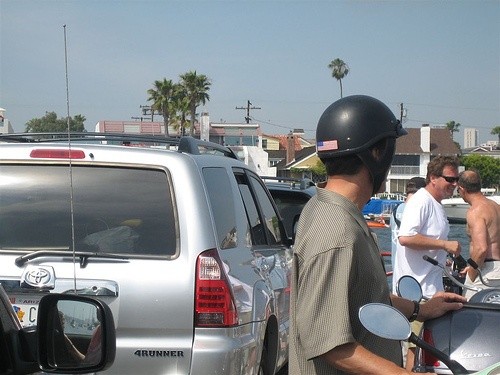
[{"x1": 0, "y1": 284, "x2": 116, "y2": 375}]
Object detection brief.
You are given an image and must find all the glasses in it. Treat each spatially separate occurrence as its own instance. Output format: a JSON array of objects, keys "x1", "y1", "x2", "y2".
[
  {"x1": 457, "y1": 187, "x2": 460, "y2": 194},
  {"x1": 439, "y1": 175, "x2": 460, "y2": 183}
]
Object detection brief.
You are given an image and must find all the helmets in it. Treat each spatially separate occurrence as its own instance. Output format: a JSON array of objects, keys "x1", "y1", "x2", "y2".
[
  {"x1": 316, "y1": 95, "x2": 408, "y2": 157},
  {"x1": 406, "y1": 177, "x2": 426, "y2": 194}
]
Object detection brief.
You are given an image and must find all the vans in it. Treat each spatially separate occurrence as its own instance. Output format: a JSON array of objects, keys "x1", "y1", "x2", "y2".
[{"x1": 363, "y1": 199, "x2": 405, "y2": 223}]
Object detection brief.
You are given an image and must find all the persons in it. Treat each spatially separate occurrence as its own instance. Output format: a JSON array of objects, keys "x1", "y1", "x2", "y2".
[
  {"x1": 386, "y1": 176, "x2": 425, "y2": 369},
  {"x1": 455, "y1": 169, "x2": 500, "y2": 302},
  {"x1": 288, "y1": 93, "x2": 467, "y2": 375},
  {"x1": 390, "y1": 155, "x2": 460, "y2": 375}
]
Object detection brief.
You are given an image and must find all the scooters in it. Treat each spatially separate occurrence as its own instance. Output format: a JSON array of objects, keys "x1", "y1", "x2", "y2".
[
  {"x1": 396, "y1": 250, "x2": 500, "y2": 303},
  {"x1": 358, "y1": 299, "x2": 500, "y2": 375}
]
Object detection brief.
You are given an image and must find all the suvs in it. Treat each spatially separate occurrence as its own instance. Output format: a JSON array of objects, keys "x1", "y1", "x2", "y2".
[
  {"x1": 0, "y1": 132, "x2": 302, "y2": 375},
  {"x1": 260, "y1": 176, "x2": 318, "y2": 247}
]
